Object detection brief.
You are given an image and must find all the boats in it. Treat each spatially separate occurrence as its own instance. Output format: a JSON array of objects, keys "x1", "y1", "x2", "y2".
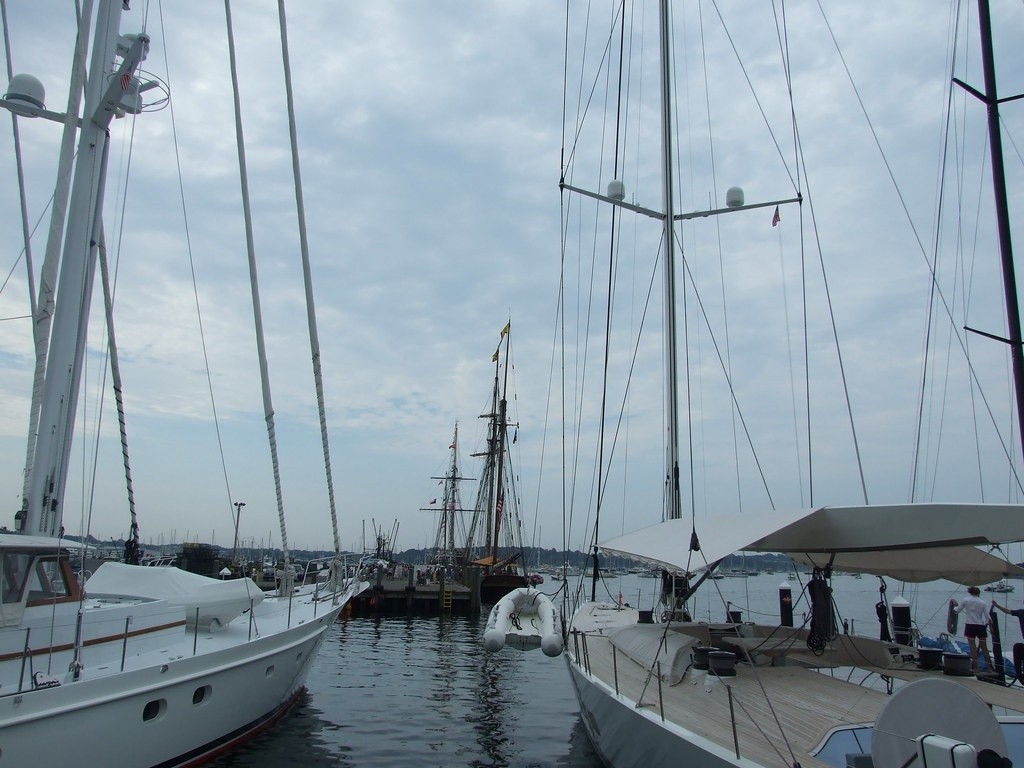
[
  {"x1": 537, "y1": 559, "x2": 663, "y2": 578},
  {"x1": 90, "y1": 317, "x2": 543, "y2": 613},
  {"x1": 787, "y1": 571, "x2": 798, "y2": 580},
  {"x1": 725, "y1": 568, "x2": 748, "y2": 577},
  {"x1": 707, "y1": 571, "x2": 724, "y2": 579},
  {"x1": 550, "y1": 568, "x2": 568, "y2": 581},
  {"x1": 483, "y1": 586, "x2": 565, "y2": 657},
  {"x1": 983, "y1": 577, "x2": 1016, "y2": 593},
  {"x1": 748, "y1": 572, "x2": 759, "y2": 576},
  {"x1": 82, "y1": 560, "x2": 265, "y2": 630}
]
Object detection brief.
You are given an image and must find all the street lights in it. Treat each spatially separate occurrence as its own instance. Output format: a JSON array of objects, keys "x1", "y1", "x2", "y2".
[{"x1": 232, "y1": 502, "x2": 246, "y2": 561}]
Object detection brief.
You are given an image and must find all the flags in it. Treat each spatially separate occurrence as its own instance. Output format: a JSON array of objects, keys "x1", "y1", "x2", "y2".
[
  {"x1": 448, "y1": 442, "x2": 456, "y2": 449},
  {"x1": 492, "y1": 351, "x2": 498, "y2": 362},
  {"x1": 501, "y1": 323, "x2": 510, "y2": 341},
  {"x1": 497, "y1": 490, "x2": 504, "y2": 513}
]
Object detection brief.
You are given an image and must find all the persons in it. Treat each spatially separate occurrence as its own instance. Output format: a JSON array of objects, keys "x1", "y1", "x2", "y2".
[
  {"x1": 416, "y1": 566, "x2": 437, "y2": 587},
  {"x1": 991, "y1": 599, "x2": 1024, "y2": 639},
  {"x1": 953, "y1": 586, "x2": 993, "y2": 676}
]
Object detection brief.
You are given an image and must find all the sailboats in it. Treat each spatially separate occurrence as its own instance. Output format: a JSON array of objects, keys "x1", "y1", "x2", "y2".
[
  {"x1": 549, "y1": 1, "x2": 1024, "y2": 768},
  {"x1": 1, "y1": 0, "x2": 371, "y2": 768}
]
